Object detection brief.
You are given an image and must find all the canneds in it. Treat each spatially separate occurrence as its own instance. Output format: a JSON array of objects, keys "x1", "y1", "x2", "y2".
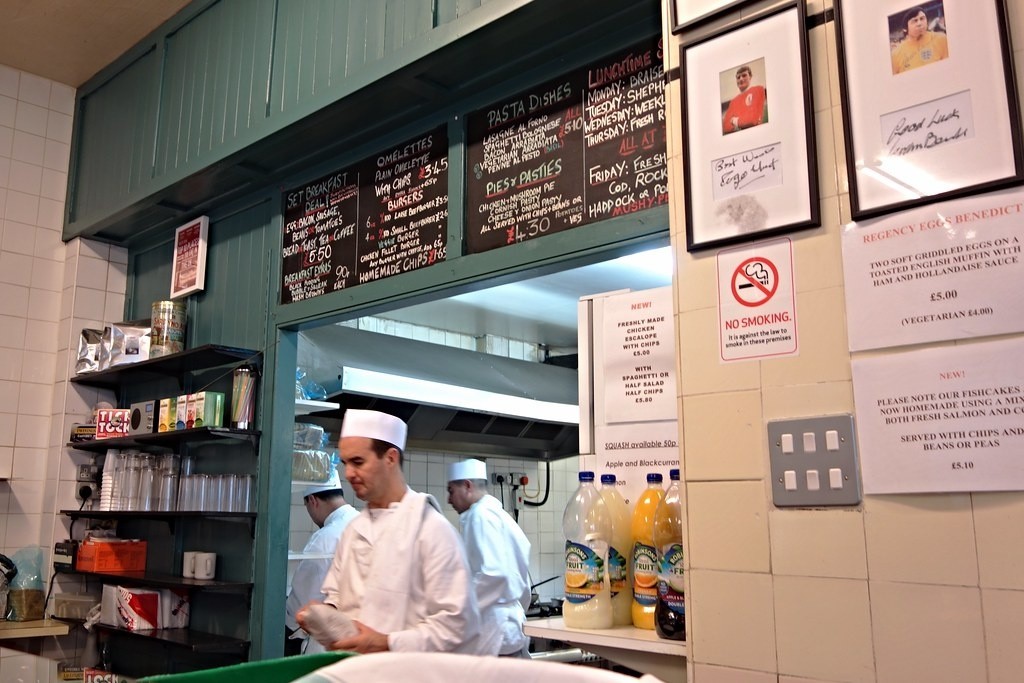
[{"x1": 148, "y1": 300, "x2": 186, "y2": 359}]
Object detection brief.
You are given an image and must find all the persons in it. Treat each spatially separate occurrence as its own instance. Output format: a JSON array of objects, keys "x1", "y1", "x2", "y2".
[
  {"x1": 295, "y1": 408, "x2": 481, "y2": 655},
  {"x1": 890, "y1": 7, "x2": 948, "y2": 76},
  {"x1": 445, "y1": 458, "x2": 532, "y2": 659},
  {"x1": 722, "y1": 66, "x2": 766, "y2": 134},
  {"x1": 285, "y1": 469, "x2": 360, "y2": 654}
]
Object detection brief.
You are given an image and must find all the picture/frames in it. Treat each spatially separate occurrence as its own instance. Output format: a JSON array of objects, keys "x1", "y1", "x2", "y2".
[
  {"x1": 668, "y1": 0, "x2": 820, "y2": 253},
  {"x1": 834, "y1": 0, "x2": 1024, "y2": 224}
]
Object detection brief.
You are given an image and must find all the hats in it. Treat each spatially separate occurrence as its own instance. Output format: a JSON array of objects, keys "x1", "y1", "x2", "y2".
[
  {"x1": 302, "y1": 470, "x2": 343, "y2": 498},
  {"x1": 446, "y1": 458, "x2": 487, "y2": 483},
  {"x1": 340, "y1": 409, "x2": 408, "y2": 452}
]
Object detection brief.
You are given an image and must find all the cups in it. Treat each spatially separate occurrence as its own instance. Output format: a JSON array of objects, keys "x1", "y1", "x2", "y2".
[
  {"x1": 183, "y1": 552, "x2": 202, "y2": 578},
  {"x1": 98, "y1": 448, "x2": 255, "y2": 512},
  {"x1": 194, "y1": 553, "x2": 216, "y2": 579}
]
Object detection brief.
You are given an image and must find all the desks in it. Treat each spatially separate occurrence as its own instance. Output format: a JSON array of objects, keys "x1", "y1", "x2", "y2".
[
  {"x1": 0, "y1": 618, "x2": 70, "y2": 656},
  {"x1": 522, "y1": 618, "x2": 688, "y2": 683}
]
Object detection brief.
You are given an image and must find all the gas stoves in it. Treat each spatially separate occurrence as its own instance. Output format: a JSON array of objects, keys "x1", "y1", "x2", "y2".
[{"x1": 525, "y1": 603, "x2": 562, "y2": 617}]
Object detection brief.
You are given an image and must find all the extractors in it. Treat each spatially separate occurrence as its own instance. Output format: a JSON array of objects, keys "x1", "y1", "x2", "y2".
[{"x1": 293, "y1": 324, "x2": 579, "y2": 463}]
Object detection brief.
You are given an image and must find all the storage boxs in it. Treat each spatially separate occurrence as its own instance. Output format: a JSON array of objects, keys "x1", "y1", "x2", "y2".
[
  {"x1": 70, "y1": 423, "x2": 96, "y2": 442},
  {"x1": 55, "y1": 592, "x2": 100, "y2": 619},
  {"x1": 158, "y1": 391, "x2": 225, "y2": 433},
  {"x1": 76, "y1": 538, "x2": 148, "y2": 572},
  {"x1": 96, "y1": 409, "x2": 130, "y2": 439}
]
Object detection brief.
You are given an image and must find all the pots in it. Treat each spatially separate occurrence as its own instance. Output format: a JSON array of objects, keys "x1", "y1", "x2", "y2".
[{"x1": 531, "y1": 593, "x2": 539, "y2": 603}]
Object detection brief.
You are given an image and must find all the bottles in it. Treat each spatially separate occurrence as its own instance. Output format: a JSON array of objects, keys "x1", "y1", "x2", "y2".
[
  {"x1": 562, "y1": 471, "x2": 613, "y2": 630},
  {"x1": 653, "y1": 469, "x2": 686, "y2": 643},
  {"x1": 591, "y1": 475, "x2": 632, "y2": 627},
  {"x1": 94, "y1": 641, "x2": 112, "y2": 673},
  {"x1": 229, "y1": 369, "x2": 256, "y2": 429},
  {"x1": 79, "y1": 626, "x2": 99, "y2": 671},
  {"x1": 630, "y1": 473, "x2": 667, "y2": 629},
  {"x1": 149, "y1": 300, "x2": 187, "y2": 359}
]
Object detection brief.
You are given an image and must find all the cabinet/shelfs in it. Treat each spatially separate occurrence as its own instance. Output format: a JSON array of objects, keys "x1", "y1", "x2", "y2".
[{"x1": 51, "y1": 344, "x2": 264, "y2": 679}]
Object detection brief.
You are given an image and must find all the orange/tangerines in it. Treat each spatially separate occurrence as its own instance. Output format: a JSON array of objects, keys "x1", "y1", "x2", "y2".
[
  {"x1": 634, "y1": 573, "x2": 659, "y2": 588},
  {"x1": 566, "y1": 572, "x2": 589, "y2": 587}
]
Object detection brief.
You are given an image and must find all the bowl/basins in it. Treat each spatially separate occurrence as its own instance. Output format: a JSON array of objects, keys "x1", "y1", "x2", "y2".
[{"x1": 55, "y1": 592, "x2": 100, "y2": 619}]
